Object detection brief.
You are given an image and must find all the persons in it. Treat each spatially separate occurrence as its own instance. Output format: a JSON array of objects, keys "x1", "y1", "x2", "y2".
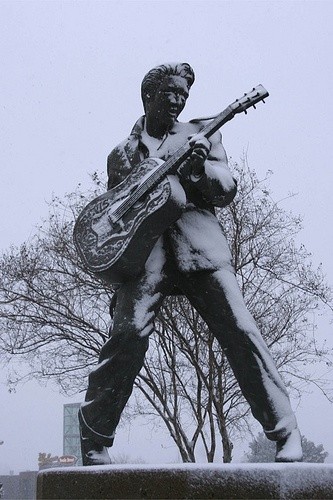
[{"x1": 76, "y1": 62, "x2": 304, "y2": 460}]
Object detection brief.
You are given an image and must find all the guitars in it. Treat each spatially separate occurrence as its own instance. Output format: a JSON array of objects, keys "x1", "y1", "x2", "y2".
[{"x1": 72, "y1": 83, "x2": 270, "y2": 282}]
